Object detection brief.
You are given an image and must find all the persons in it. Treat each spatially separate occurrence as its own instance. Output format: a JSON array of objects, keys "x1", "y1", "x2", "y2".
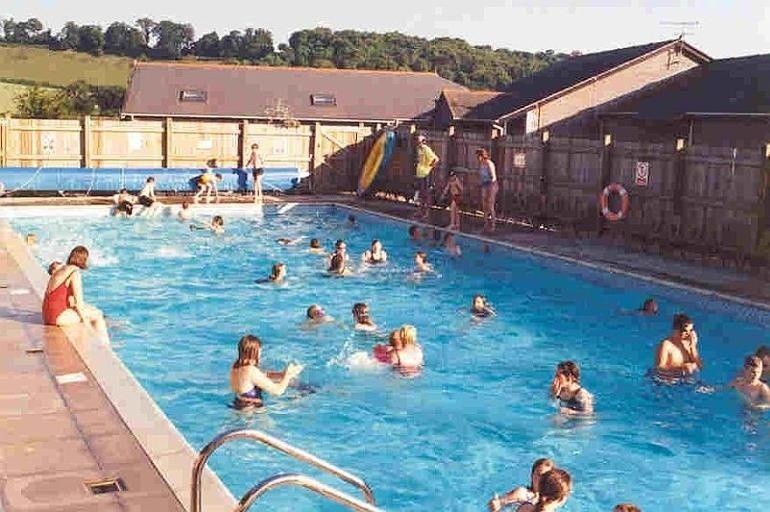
[
  {"x1": 245, "y1": 144, "x2": 263, "y2": 199},
  {"x1": 118, "y1": 176, "x2": 154, "y2": 218},
  {"x1": 195, "y1": 174, "x2": 222, "y2": 197},
  {"x1": 178, "y1": 201, "x2": 496, "y2": 411},
  {"x1": 42, "y1": 244, "x2": 104, "y2": 328},
  {"x1": 415, "y1": 133, "x2": 499, "y2": 235},
  {"x1": 489, "y1": 298, "x2": 769, "y2": 512}
]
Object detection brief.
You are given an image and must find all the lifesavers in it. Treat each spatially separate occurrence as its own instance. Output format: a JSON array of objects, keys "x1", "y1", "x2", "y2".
[
  {"x1": 358, "y1": 130, "x2": 395, "y2": 198},
  {"x1": 601, "y1": 183, "x2": 630, "y2": 221}
]
